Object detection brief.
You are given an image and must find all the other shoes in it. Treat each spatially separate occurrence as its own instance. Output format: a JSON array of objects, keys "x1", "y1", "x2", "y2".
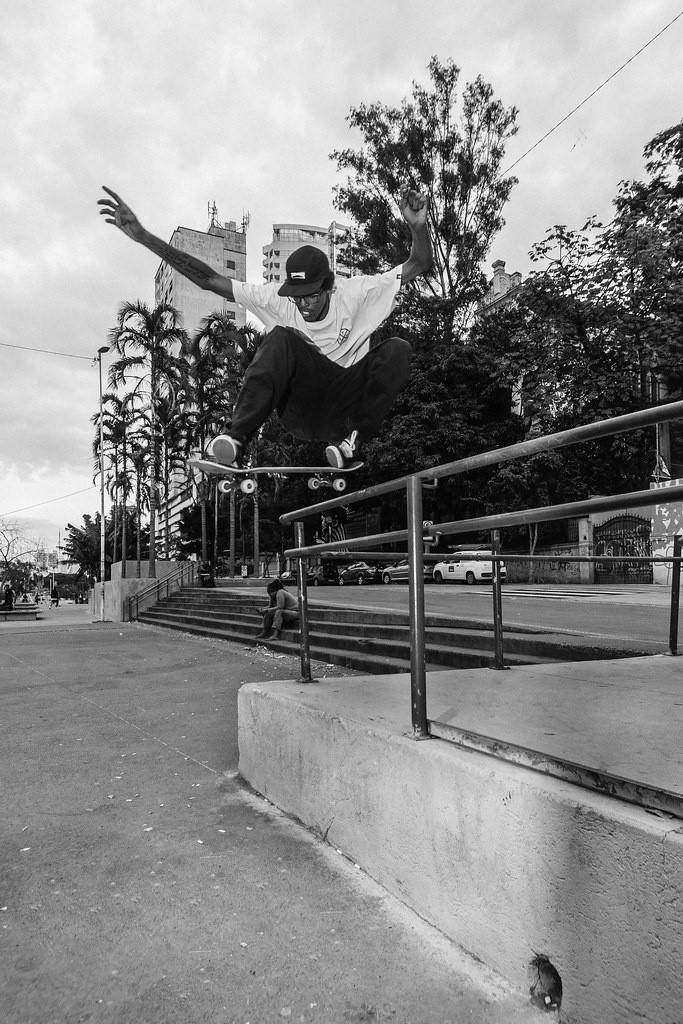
[
  {"x1": 255, "y1": 634, "x2": 269, "y2": 638},
  {"x1": 265, "y1": 636, "x2": 279, "y2": 641}
]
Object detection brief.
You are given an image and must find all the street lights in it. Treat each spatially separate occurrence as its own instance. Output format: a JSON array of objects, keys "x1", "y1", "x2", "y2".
[{"x1": 96, "y1": 344, "x2": 112, "y2": 623}]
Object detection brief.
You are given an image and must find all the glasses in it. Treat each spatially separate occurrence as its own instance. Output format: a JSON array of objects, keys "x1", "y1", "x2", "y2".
[{"x1": 287, "y1": 285, "x2": 325, "y2": 304}]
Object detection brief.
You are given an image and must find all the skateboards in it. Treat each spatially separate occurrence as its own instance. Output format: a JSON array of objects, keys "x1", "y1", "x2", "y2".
[{"x1": 186, "y1": 458, "x2": 363, "y2": 494}]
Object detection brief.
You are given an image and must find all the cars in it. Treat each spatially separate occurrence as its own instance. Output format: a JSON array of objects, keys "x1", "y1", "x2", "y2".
[
  {"x1": 382, "y1": 557, "x2": 437, "y2": 584},
  {"x1": 339, "y1": 560, "x2": 387, "y2": 585},
  {"x1": 433, "y1": 549, "x2": 508, "y2": 585},
  {"x1": 305, "y1": 564, "x2": 339, "y2": 586}
]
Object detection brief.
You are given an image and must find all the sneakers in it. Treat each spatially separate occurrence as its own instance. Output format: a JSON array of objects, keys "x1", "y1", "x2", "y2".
[
  {"x1": 326, "y1": 430, "x2": 359, "y2": 469},
  {"x1": 211, "y1": 433, "x2": 246, "y2": 469}
]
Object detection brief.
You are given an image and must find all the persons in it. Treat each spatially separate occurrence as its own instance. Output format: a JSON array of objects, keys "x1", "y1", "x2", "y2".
[
  {"x1": 197, "y1": 560, "x2": 216, "y2": 587},
  {"x1": 1, "y1": 584, "x2": 87, "y2": 611},
  {"x1": 97, "y1": 183, "x2": 436, "y2": 467},
  {"x1": 254, "y1": 580, "x2": 300, "y2": 643}
]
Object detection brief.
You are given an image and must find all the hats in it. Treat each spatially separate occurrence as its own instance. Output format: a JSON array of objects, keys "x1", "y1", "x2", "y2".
[{"x1": 278, "y1": 245, "x2": 331, "y2": 296}]
